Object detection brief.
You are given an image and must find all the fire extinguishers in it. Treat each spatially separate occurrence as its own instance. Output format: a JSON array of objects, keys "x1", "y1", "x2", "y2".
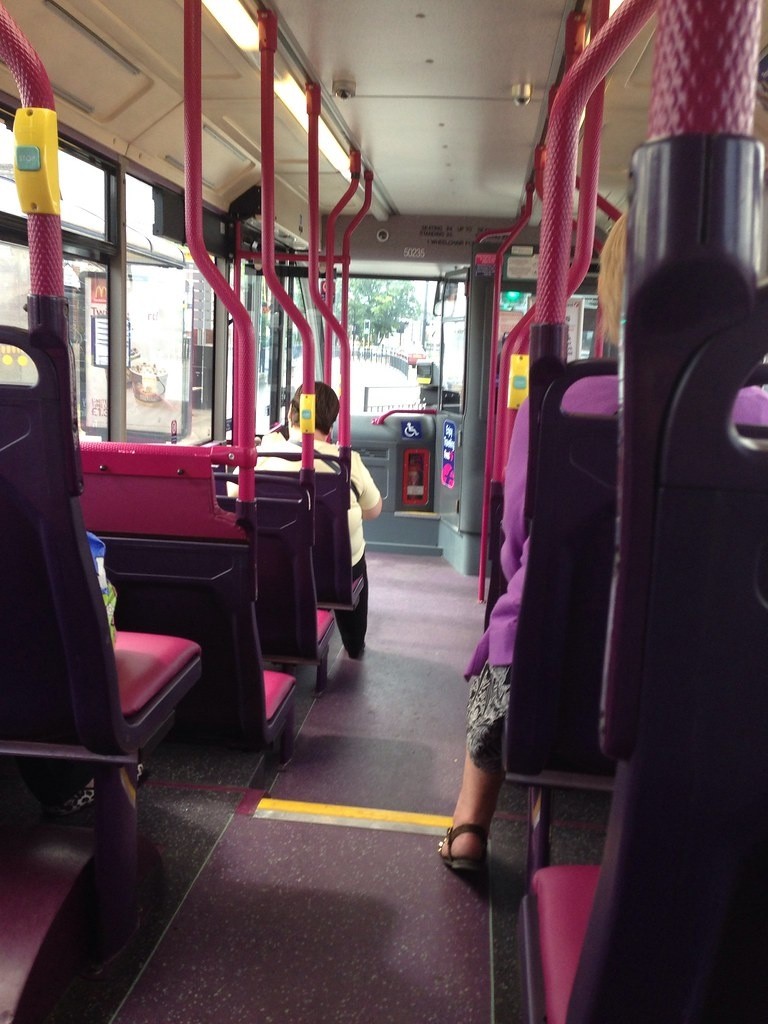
[{"x1": 407, "y1": 456, "x2": 422, "y2": 500}]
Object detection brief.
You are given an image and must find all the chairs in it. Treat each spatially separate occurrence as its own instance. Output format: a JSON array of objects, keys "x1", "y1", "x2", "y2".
[
  {"x1": 0, "y1": 325, "x2": 203, "y2": 943},
  {"x1": 483, "y1": 282, "x2": 768, "y2": 1024}
]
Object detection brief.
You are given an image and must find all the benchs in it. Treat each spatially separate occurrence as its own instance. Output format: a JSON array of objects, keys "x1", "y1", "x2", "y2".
[
  {"x1": 211, "y1": 450, "x2": 366, "y2": 612},
  {"x1": 78, "y1": 442, "x2": 300, "y2": 774}
]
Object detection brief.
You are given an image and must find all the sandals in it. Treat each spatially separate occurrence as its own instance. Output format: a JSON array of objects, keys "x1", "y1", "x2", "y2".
[{"x1": 438, "y1": 823, "x2": 487, "y2": 871}]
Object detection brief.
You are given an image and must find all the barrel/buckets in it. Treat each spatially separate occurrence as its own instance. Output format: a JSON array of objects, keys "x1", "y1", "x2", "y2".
[{"x1": 105, "y1": 349, "x2": 169, "y2": 406}]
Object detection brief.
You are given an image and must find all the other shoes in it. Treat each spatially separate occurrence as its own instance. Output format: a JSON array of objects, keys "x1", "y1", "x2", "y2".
[
  {"x1": 349, "y1": 642, "x2": 365, "y2": 658},
  {"x1": 42, "y1": 762, "x2": 149, "y2": 824}
]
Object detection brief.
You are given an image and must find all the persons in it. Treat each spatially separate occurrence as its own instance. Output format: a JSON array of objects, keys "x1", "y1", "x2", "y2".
[
  {"x1": 437, "y1": 214, "x2": 768, "y2": 870},
  {"x1": 226, "y1": 381, "x2": 382, "y2": 657},
  {"x1": 434, "y1": 285, "x2": 456, "y2": 317}
]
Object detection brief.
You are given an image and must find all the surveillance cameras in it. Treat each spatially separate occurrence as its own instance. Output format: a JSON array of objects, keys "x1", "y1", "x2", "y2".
[
  {"x1": 376, "y1": 229, "x2": 388, "y2": 242},
  {"x1": 511, "y1": 84, "x2": 531, "y2": 107},
  {"x1": 331, "y1": 79, "x2": 356, "y2": 100}
]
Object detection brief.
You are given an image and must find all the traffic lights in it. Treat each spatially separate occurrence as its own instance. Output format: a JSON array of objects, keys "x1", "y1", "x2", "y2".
[{"x1": 501, "y1": 288, "x2": 528, "y2": 306}]
